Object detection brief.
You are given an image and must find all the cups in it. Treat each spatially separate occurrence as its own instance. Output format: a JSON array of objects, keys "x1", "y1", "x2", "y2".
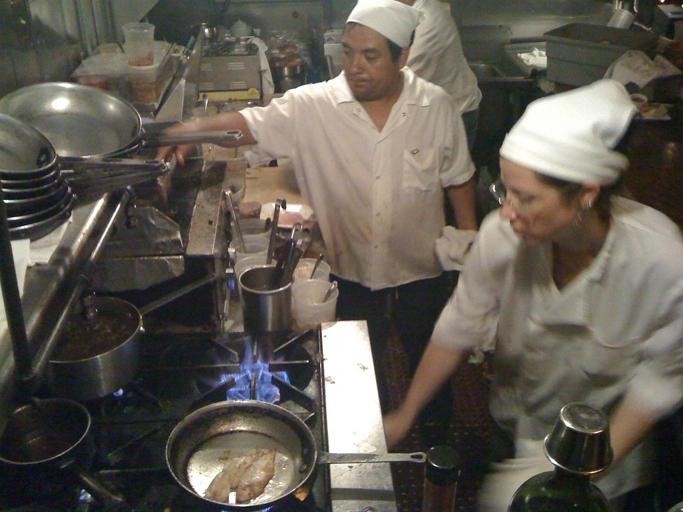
[
  {"x1": 238, "y1": 259, "x2": 340, "y2": 329},
  {"x1": 544, "y1": 403, "x2": 612, "y2": 475},
  {"x1": 122, "y1": 22, "x2": 155, "y2": 65},
  {"x1": 630, "y1": 94, "x2": 647, "y2": 114}
]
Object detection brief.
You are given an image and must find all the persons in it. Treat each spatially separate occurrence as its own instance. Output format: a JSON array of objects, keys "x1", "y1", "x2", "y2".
[
  {"x1": 381, "y1": 79, "x2": 683, "y2": 512},
  {"x1": 156, "y1": 0, "x2": 476, "y2": 450},
  {"x1": 397, "y1": 0, "x2": 483, "y2": 154}
]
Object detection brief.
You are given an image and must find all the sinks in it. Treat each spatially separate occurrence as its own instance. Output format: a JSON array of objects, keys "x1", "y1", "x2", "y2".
[{"x1": 469, "y1": 62, "x2": 510, "y2": 117}]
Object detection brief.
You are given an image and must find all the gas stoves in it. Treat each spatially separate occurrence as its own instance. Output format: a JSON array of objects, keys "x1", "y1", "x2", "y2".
[{"x1": 47, "y1": 331, "x2": 329, "y2": 512}]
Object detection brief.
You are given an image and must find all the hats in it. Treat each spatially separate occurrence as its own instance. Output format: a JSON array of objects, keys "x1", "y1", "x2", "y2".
[
  {"x1": 498, "y1": 78, "x2": 639, "y2": 187},
  {"x1": 345, "y1": 1, "x2": 423, "y2": 49}
]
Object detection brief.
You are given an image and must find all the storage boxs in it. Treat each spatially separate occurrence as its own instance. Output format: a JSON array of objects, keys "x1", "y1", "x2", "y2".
[{"x1": 544, "y1": 23, "x2": 660, "y2": 89}]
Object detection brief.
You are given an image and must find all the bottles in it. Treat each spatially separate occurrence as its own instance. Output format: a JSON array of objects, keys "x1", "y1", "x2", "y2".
[
  {"x1": 505, "y1": 465, "x2": 610, "y2": 512},
  {"x1": 422, "y1": 446, "x2": 462, "y2": 512}
]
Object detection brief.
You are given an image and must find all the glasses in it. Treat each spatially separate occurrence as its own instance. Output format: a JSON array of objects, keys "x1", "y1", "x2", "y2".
[{"x1": 487, "y1": 184, "x2": 546, "y2": 214}]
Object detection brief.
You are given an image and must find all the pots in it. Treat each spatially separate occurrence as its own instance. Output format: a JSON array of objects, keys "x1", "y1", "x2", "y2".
[
  {"x1": 43, "y1": 271, "x2": 226, "y2": 399},
  {"x1": 166, "y1": 399, "x2": 427, "y2": 508},
  {"x1": 1, "y1": 82, "x2": 243, "y2": 242},
  {"x1": 1, "y1": 400, "x2": 123, "y2": 510}
]
